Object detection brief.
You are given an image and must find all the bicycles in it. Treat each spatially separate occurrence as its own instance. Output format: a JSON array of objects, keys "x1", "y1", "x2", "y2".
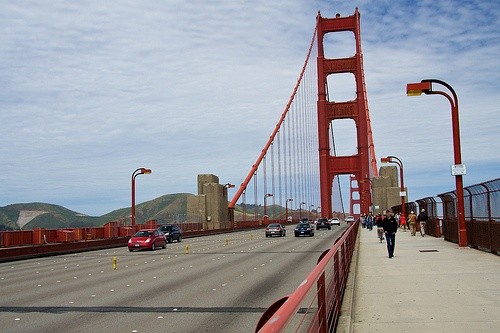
[{"x1": 376, "y1": 228, "x2": 384, "y2": 244}]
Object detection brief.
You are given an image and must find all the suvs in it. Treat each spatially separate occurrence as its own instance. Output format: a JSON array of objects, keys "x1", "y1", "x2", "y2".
[{"x1": 345, "y1": 216, "x2": 355, "y2": 224}]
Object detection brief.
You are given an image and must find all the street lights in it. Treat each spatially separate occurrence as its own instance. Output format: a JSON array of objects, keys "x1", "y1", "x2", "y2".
[
  {"x1": 406, "y1": 78, "x2": 469, "y2": 246},
  {"x1": 299, "y1": 202, "x2": 321, "y2": 219},
  {"x1": 285, "y1": 198, "x2": 293, "y2": 224},
  {"x1": 381, "y1": 155, "x2": 406, "y2": 226},
  {"x1": 264, "y1": 193, "x2": 274, "y2": 215},
  {"x1": 130, "y1": 167, "x2": 152, "y2": 225}
]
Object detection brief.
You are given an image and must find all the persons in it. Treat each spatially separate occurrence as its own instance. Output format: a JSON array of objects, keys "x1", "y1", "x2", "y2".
[
  {"x1": 359, "y1": 206, "x2": 429, "y2": 236},
  {"x1": 382, "y1": 210, "x2": 398, "y2": 259}
]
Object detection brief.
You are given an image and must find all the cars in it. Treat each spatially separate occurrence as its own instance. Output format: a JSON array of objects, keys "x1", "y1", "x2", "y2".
[
  {"x1": 265, "y1": 223, "x2": 286, "y2": 238},
  {"x1": 294, "y1": 223, "x2": 315, "y2": 237},
  {"x1": 128, "y1": 229, "x2": 167, "y2": 251},
  {"x1": 297, "y1": 216, "x2": 340, "y2": 230},
  {"x1": 156, "y1": 224, "x2": 183, "y2": 243}
]
